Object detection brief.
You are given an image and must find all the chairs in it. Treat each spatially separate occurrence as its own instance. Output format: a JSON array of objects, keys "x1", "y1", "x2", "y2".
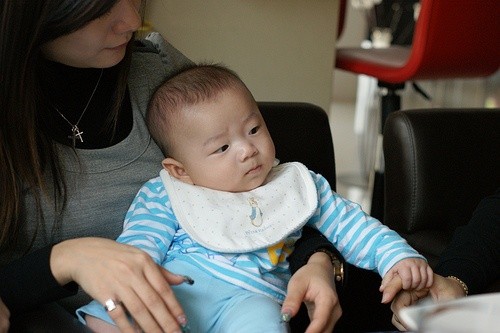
[{"x1": 257, "y1": 0, "x2": 500, "y2": 333}]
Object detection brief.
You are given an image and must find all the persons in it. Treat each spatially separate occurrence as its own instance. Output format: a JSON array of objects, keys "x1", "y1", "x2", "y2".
[
  {"x1": 390, "y1": 193, "x2": 500, "y2": 333},
  {"x1": 75, "y1": 60, "x2": 434, "y2": 333},
  {"x1": 0, "y1": 0, "x2": 343, "y2": 333}
]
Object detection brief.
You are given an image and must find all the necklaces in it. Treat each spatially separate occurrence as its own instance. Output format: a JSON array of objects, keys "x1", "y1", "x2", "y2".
[{"x1": 53, "y1": 67, "x2": 104, "y2": 148}]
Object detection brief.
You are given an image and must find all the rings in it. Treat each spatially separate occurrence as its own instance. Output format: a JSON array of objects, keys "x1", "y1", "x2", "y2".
[
  {"x1": 104, "y1": 299, "x2": 116, "y2": 312},
  {"x1": 410, "y1": 291, "x2": 418, "y2": 299}
]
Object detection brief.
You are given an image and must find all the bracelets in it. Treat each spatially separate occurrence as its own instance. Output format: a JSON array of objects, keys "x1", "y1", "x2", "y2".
[{"x1": 448, "y1": 276, "x2": 468, "y2": 296}]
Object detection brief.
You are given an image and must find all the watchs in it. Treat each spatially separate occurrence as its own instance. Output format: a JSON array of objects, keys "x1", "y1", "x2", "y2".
[{"x1": 314, "y1": 249, "x2": 344, "y2": 281}]
